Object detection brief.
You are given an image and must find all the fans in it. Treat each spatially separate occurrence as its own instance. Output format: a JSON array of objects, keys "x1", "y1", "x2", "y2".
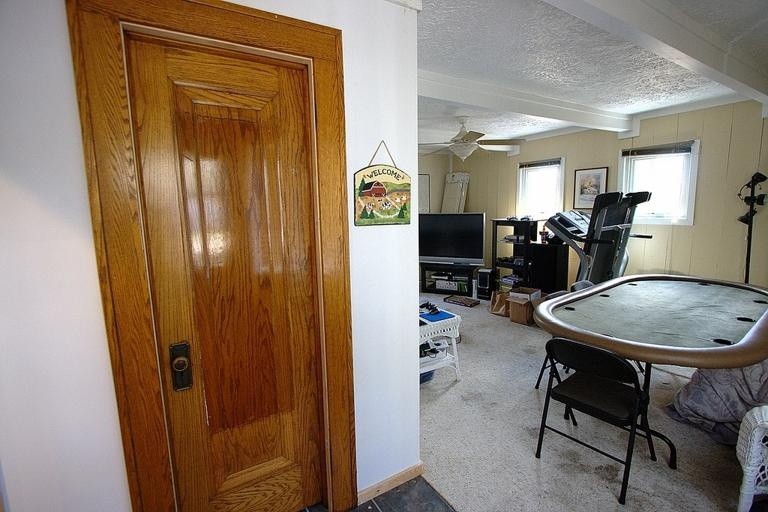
[{"x1": 418, "y1": 115, "x2": 527, "y2": 157}]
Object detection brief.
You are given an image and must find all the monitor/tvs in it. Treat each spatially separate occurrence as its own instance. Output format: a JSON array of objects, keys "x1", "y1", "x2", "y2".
[{"x1": 419, "y1": 212, "x2": 485, "y2": 265}]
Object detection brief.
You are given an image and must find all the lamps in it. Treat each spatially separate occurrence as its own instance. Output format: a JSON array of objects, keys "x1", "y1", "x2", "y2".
[{"x1": 448, "y1": 142, "x2": 479, "y2": 162}]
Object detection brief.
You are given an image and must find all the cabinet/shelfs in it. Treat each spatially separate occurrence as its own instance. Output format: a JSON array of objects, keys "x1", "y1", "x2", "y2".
[
  {"x1": 490, "y1": 218, "x2": 569, "y2": 292},
  {"x1": 421, "y1": 264, "x2": 473, "y2": 297}
]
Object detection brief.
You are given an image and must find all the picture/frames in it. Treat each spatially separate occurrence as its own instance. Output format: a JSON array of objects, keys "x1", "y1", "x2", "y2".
[{"x1": 573, "y1": 167, "x2": 608, "y2": 209}]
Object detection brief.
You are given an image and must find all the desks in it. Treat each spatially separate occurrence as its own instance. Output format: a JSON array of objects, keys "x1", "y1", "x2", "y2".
[{"x1": 532, "y1": 273, "x2": 768, "y2": 471}]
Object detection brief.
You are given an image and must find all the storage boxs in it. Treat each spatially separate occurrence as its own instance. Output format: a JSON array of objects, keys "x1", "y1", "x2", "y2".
[{"x1": 504, "y1": 286, "x2": 548, "y2": 326}]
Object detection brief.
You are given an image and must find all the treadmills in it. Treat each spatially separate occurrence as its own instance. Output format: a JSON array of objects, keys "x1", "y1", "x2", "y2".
[{"x1": 545, "y1": 191, "x2": 653, "y2": 284}]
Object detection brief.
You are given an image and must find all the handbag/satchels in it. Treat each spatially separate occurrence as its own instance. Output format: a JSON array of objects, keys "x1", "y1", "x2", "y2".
[{"x1": 489, "y1": 291, "x2": 510, "y2": 317}]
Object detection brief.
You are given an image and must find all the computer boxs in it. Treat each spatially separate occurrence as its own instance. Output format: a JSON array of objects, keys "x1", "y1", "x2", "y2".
[{"x1": 477, "y1": 268, "x2": 492, "y2": 301}]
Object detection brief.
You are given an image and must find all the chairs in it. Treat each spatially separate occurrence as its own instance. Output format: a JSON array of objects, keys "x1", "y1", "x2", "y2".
[{"x1": 535, "y1": 337, "x2": 657, "y2": 505}]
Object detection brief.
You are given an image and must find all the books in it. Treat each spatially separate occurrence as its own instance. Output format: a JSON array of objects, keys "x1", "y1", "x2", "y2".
[{"x1": 443, "y1": 294, "x2": 481, "y2": 308}]
